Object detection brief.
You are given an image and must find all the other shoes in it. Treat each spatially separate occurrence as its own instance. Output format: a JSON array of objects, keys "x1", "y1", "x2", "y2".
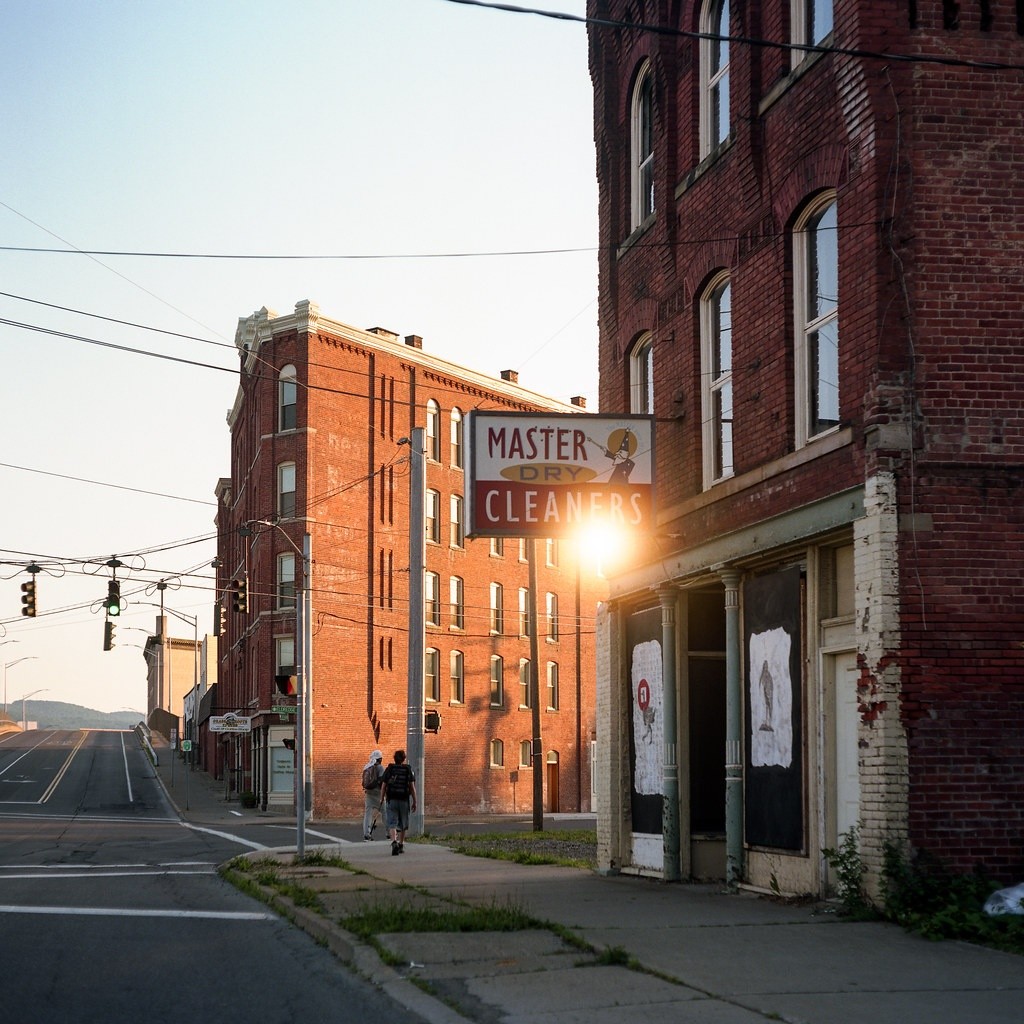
[
  {"x1": 391, "y1": 841, "x2": 399, "y2": 856},
  {"x1": 364, "y1": 836, "x2": 375, "y2": 841},
  {"x1": 386, "y1": 834, "x2": 399, "y2": 841},
  {"x1": 398, "y1": 843, "x2": 403, "y2": 853}
]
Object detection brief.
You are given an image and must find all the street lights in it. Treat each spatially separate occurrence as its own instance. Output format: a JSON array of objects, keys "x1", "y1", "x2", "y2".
[
  {"x1": 5, "y1": 657, "x2": 39, "y2": 713},
  {"x1": 23, "y1": 689, "x2": 50, "y2": 730},
  {"x1": 122, "y1": 601, "x2": 198, "y2": 771},
  {"x1": 258, "y1": 520, "x2": 313, "y2": 856}
]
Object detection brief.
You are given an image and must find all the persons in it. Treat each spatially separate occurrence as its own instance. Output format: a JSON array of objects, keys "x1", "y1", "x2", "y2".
[
  {"x1": 379, "y1": 750, "x2": 416, "y2": 855},
  {"x1": 361, "y1": 750, "x2": 391, "y2": 842}
]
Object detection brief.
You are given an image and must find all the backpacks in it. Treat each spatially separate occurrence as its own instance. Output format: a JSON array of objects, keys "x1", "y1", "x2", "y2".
[
  {"x1": 361, "y1": 765, "x2": 380, "y2": 789},
  {"x1": 387, "y1": 763, "x2": 410, "y2": 800}
]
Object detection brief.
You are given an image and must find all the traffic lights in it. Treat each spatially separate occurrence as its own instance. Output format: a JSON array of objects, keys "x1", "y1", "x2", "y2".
[
  {"x1": 21, "y1": 581, "x2": 37, "y2": 618},
  {"x1": 232, "y1": 579, "x2": 249, "y2": 614},
  {"x1": 104, "y1": 622, "x2": 116, "y2": 651},
  {"x1": 108, "y1": 580, "x2": 121, "y2": 616}
]
